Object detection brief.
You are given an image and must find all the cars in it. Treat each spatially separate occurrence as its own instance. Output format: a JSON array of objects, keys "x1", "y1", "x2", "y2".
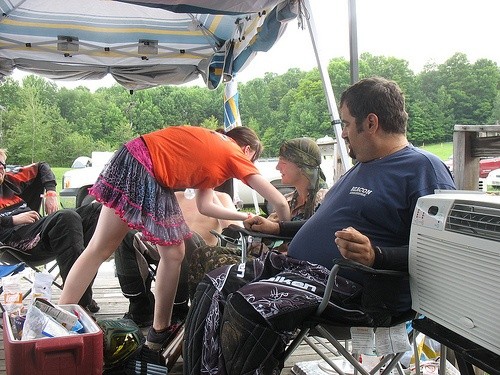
[{"x1": 442, "y1": 153, "x2": 500, "y2": 188}]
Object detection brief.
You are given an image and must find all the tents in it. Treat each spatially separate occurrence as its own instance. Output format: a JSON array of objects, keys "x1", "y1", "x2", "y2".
[{"x1": 0, "y1": 0, "x2": 358, "y2": 215}]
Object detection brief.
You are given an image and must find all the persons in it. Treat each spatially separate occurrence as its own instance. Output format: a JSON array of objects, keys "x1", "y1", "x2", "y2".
[
  {"x1": 188, "y1": 137, "x2": 330, "y2": 312},
  {"x1": 58, "y1": 125, "x2": 291, "y2": 351},
  {"x1": 183, "y1": 77, "x2": 456, "y2": 375},
  {"x1": 115, "y1": 177, "x2": 244, "y2": 328},
  {"x1": 0, "y1": 149, "x2": 103, "y2": 323}
]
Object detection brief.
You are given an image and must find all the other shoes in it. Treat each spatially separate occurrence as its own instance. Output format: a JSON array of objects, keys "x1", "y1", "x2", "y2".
[
  {"x1": 147, "y1": 324, "x2": 182, "y2": 349},
  {"x1": 82, "y1": 307, "x2": 97, "y2": 323},
  {"x1": 122, "y1": 312, "x2": 152, "y2": 326},
  {"x1": 89, "y1": 300, "x2": 101, "y2": 312}
]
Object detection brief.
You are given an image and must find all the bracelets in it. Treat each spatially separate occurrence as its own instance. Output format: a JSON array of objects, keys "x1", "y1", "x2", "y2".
[{"x1": 45, "y1": 192, "x2": 57, "y2": 196}]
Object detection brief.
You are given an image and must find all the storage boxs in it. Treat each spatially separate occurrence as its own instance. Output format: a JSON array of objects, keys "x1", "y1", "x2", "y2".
[{"x1": 3, "y1": 304, "x2": 103, "y2": 375}]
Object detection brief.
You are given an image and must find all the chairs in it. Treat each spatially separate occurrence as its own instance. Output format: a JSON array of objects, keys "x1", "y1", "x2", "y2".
[
  {"x1": 228, "y1": 224, "x2": 419, "y2": 375},
  {"x1": 0, "y1": 240, "x2": 64, "y2": 329}
]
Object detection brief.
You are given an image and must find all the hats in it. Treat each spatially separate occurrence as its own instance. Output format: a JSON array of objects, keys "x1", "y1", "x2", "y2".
[{"x1": 280, "y1": 137, "x2": 321, "y2": 168}]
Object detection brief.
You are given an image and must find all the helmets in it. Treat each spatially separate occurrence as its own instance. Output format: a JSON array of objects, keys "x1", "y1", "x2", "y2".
[{"x1": 98, "y1": 318, "x2": 145, "y2": 370}]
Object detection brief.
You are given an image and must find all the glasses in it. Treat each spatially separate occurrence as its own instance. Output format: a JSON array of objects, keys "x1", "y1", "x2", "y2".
[{"x1": 0, "y1": 162, "x2": 6, "y2": 169}]
